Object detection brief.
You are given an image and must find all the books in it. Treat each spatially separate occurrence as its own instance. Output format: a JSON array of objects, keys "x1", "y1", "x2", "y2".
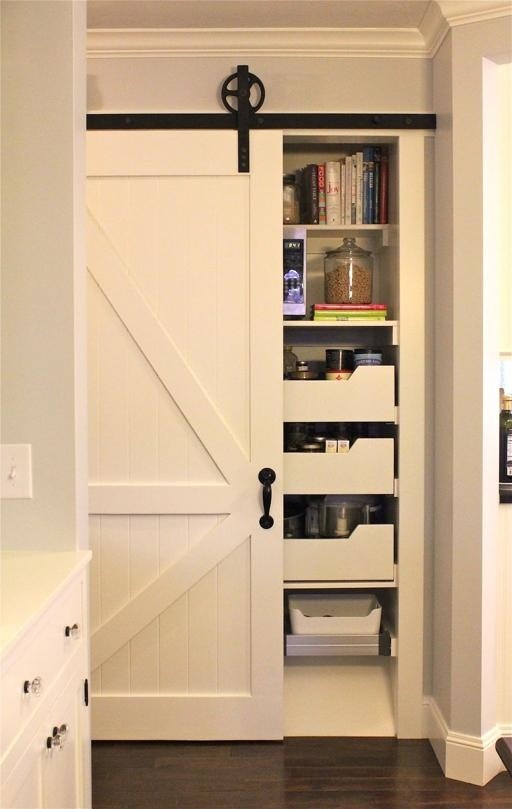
[
  {"x1": 310, "y1": 301, "x2": 390, "y2": 320},
  {"x1": 301, "y1": 146, "x2": 389, "y2": 223}
]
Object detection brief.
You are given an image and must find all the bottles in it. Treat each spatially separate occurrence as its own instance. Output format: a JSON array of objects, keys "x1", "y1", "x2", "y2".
[
  {"x1": 500, "y1": 394, "x2": 512, "y2": 482},
  {"x1": 283, "y1": 346, "x2": 298, "y2": 381},
  {"x1": 283, "y1": 174, "x2": 303, "y2": 224}
]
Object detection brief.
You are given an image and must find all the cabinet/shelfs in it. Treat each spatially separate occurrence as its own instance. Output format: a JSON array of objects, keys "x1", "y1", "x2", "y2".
[{"x1": 281, "y1": 131, "x2": 425, "y2": 739}]
